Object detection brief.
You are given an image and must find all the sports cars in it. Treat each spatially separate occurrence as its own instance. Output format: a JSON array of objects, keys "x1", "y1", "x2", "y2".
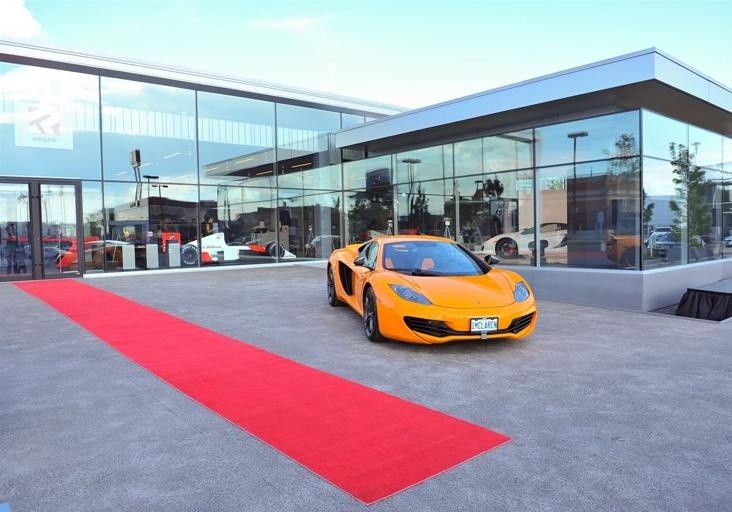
[
  {"x1": 325, "y1": 230, "x2": 536, "y2": 346},
  {"x1": 479, "y1": 220, "x2": 569, "y2": 260}
]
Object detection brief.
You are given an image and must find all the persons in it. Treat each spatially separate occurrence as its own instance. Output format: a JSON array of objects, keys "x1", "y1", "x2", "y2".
[
  {"x1": 489, "y1": 208, "x2": 503, "y2": 239},
  {"x1": 189, "y1": 218, "x2": 198, "y2": 240},
  {"x1": 203, "y1": 215, "x2": 216, "y2": 235}
]
